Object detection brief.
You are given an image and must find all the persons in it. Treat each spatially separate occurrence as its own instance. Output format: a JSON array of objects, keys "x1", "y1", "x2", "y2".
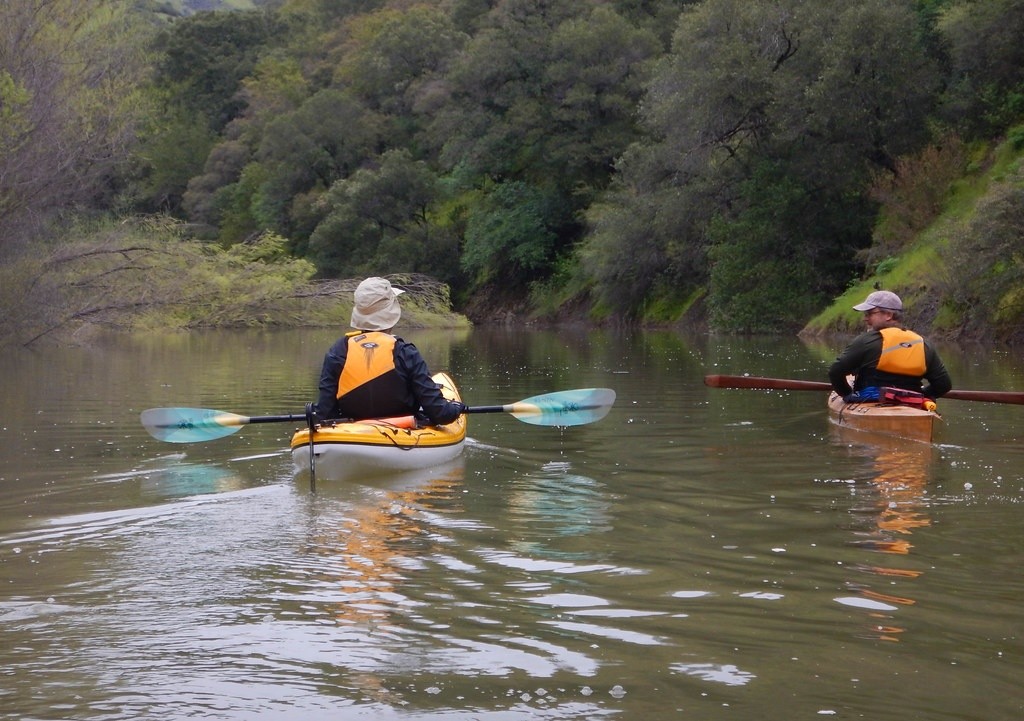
[
  {"x1": 829, "y1": 290, "x2": 952, "y2": 403},
  {"x1": 317, "y1": 276, "x2": 464, "y2": 426}
]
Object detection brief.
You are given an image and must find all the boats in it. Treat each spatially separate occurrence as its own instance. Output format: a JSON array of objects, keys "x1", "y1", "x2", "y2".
[
  {"x1": 289, "y1": 372, "x2": 466, "y2": 482},
  {"x1": 828, "y1": 374, "x2": 935, "y2": 447}
]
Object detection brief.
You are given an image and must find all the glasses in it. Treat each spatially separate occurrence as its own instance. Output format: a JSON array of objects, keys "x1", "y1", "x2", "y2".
[{"x1": 864, "y1": 311, "x2": 882, "y2": 317}]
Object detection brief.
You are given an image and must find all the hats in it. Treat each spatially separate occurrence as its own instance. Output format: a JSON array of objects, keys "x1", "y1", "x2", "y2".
[
  {"x1": 351, "y1": 276, "x2": 405, "y2": 331},
  {"x1": 852, "y1": 291, "x2": 903, "y2": 311}
]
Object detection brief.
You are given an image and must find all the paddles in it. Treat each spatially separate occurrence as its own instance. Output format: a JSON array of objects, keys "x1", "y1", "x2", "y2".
[
  {"x1": 703, "y1": 374, "x2": 1024, "y2": 405},
  {"x1": 139, "y1": 387, "x2": 617, "y2": 444}
]
